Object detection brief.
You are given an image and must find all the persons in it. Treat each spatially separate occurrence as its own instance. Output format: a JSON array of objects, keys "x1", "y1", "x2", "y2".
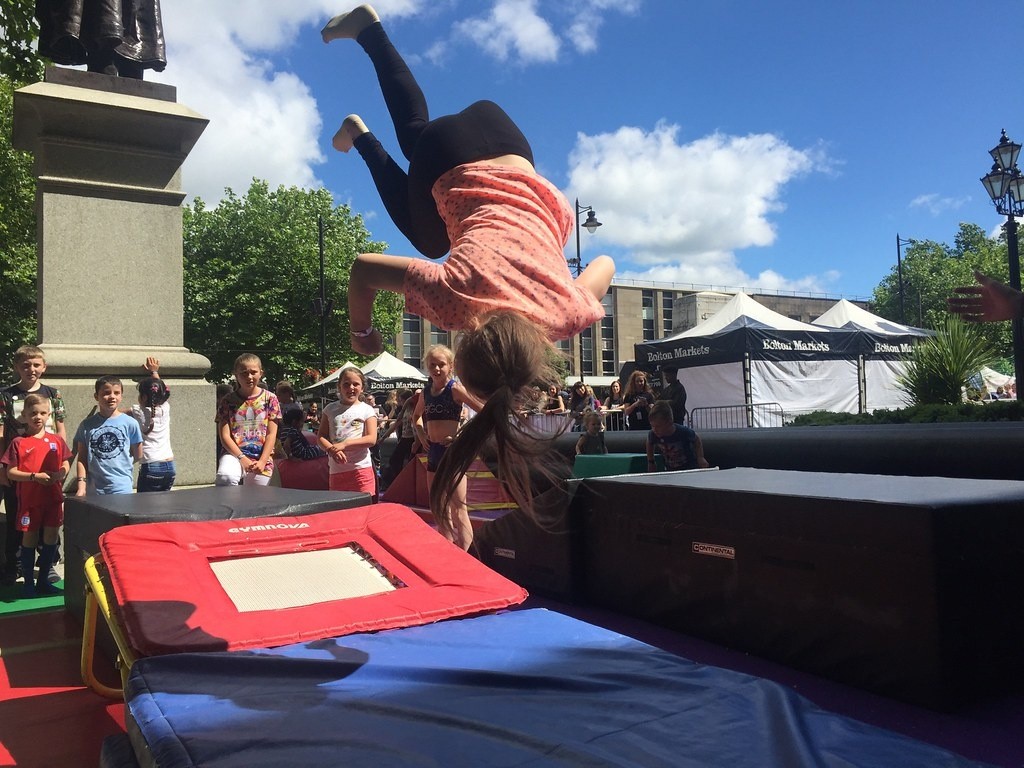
[
  {"x1": 320, "y1": 4, "x2": 617, "y2": 542},
  {"x1": 530, "y1": 369, "x2": 711, "y2": 473},
  {"x1": 214, "y1": 342, "x2": 495, "y2": 563},
  {"x1": 989, "y1": 377, "x2": 1018, "y2": 401},
  {"x1": 118, "y1": 357, "x2": 177, "y2": 492},
  {"x1": 0, "y1": 343, "x2": 67, "y2": 584},
  {"x1": 1, "y1": 393, "x2": 73, "y2": 596},
  {"x1": 76, "y1": 375, "x2": 143, "y2": 497}
]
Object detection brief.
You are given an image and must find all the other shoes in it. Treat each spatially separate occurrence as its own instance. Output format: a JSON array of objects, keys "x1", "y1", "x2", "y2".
[
  {"x1": 1, "y1": 557, "x2": 24, "y2": 585},
  {"x1": 21, "y1": 582, "x2": 37, "y2": 597},
  {"x1": 40, "y1": 564, "x2": 61, "y2": 584},
  {"x1": 35, "y1": 582, "x2": 61, "y2": 596}
]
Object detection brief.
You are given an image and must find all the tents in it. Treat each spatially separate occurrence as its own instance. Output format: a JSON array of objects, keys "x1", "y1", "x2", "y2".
[
  {"x1": 634, "y1": 291, "x2": 1014, "y2": 431},
  {"x1": 358, "y1": 349, "x2": 431, "y2": 394},
  {"x1": 300, "y1": 361, "x2": 363, "y2": 412}
]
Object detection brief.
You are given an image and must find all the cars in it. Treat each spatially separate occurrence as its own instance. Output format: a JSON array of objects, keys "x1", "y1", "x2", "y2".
[{"x1": 981, "y1": 390, "x2": 1017, "y2": 404}]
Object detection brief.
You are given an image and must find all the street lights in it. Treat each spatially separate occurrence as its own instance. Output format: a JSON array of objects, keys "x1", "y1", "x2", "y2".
[
  {"x1": 576, "y1": 197, "x2": 602, "y2": 381},
  {"x1": 978, "y1": 127, "x2": 1024, "y2": 403}
]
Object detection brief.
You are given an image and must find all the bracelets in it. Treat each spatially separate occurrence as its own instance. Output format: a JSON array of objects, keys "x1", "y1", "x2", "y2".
[
  {"x1": 77, "y1": 477, "x2": 88, "y2": 482},
  {"x1": 236, "y1": 453, "x2": 245, "y2": 459},
  {"x1": 349, "y1": 324, "x2": 375, "y2": 338},
  {"x1": 648, "y1": 459, "x2": 656, "y2": 464},
  {"x1": 381, "y1": 434, "x2": 388, "y2": 438},
  {"x1": 30, "y1": 472, "x2": 36, "y2": 484}
]
